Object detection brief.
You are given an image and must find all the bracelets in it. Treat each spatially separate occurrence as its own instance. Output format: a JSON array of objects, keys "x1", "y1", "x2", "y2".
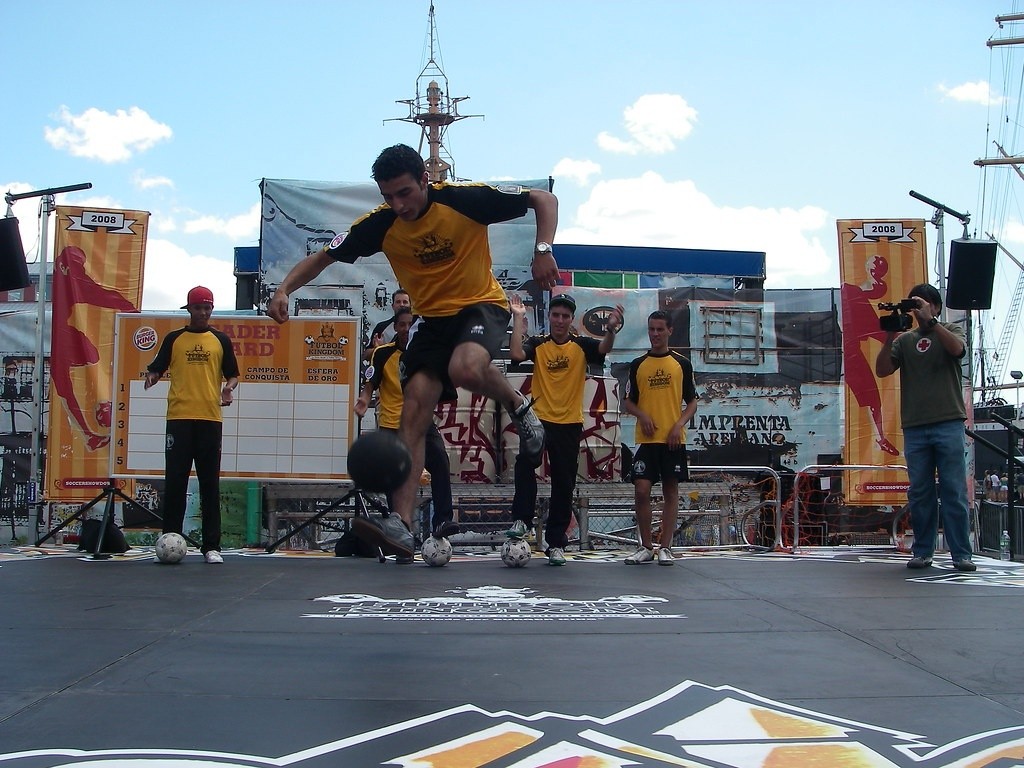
[{"x1": 607, "y1": 327, "x2": 616, "y2": 334}]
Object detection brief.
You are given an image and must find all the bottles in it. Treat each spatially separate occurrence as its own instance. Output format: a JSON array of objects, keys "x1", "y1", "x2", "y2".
[{"x1": 999, "y1": 530, "x2": 1010, "y2": 561}]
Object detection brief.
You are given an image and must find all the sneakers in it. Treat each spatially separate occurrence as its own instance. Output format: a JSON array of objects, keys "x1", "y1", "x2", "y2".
[
  {"x1": 624, "y1": 543, "x2": 658, "y2": 563},
  {"x1": 153, "y1": 554, "x2": 160, "y2": 562},
  {"x1": 509, "y1": 389, "x2": 546, "y2": 456},
  {"x1": 394, "y1": 553, "x2": 414, "y2": 565},
  {"x1": 549, "y1": 545, "x2": 566, "y2": 563},
  {"x1": 953, "y1": 558, "x2": 978, "y2": 571},
  {"x1": 351, "y1": 512, "x2": 415, "y2": 557},
  {"x1": 432, "y1": 522, "x2": 460, "y2": 536},
  {"x1": 658, "y1": 548, "x2": 673, "y2": 565},
  {"x1": 506, "y1": 519, "x2": 531, "y2": 540},
  {"x1": 204, "y1": 549, "x2": 223, "y2": 563},
  {"x1": 907, "y1": 557, "x2": 932, "y2": 568}
]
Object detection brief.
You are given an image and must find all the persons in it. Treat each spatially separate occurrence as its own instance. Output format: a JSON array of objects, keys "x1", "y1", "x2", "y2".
[
  {"x1": 352, "y1": 308, "x2": 459, "y2": 564},
  {"x1": 876, "y1": 284, "x2": 977, "y2": 571},
  {"x1": 623, "y1": 310, "x2": 697, "y2": 566},
  {"x1": 505, "y1": 293, "x2": 624, "y2": 564},
  {"x1": 983, "y1": 470, "x2": 1008, "y2": 503},
  {"x1": 365, "y1": 289, "x2": 429, "y2": 483},
  {"x1": 266, "y1": 144, "x2": 561, "y2": 558},
  {"x1": 144, "y1": 285, "x2": 240, "y2": 563}
]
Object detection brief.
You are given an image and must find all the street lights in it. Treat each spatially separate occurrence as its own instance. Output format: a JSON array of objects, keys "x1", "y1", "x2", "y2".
[{"x1": 1010, "y1": 370, "x2": 1023, "y2": 420}]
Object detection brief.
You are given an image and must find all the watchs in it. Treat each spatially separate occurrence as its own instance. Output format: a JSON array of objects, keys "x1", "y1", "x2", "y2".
[
  {"x1": 534, "y1": 241, "x2": 552, "y2": 254},
  {"x1": 928, "y1": 319, "x2": 937, "y2": 327}
]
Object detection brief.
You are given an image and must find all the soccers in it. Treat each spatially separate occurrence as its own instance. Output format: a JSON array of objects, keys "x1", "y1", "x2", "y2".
[
  {"x1": 502, "y1": 538, "x2": 532, "y2": 567},
  {"x1": 348, "y1": 431, "x2": 410, "y2": 493},
  {"x1": 422, "y1": 537, "x2": 452, "y2": 567},
  {"x1": 155, "y1": 533, "x2": 187, "y2": 563}
]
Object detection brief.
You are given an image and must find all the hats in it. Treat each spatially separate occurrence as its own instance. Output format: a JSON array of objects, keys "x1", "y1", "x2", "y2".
[
  {"x1": 180, "y1": 285, "x2": 214, "y2": 310},
  {"x1": 550, "y1": 294, "x2": 577, "y2": 311}
]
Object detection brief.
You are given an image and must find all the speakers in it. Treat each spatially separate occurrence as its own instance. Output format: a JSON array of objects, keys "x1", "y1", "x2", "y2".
[
  {"x1": 946, "y1": 237, "x2": 998, "y2": 310},
  {"x1": 0, "y1": 217, "x2": 32, "y2": 291}
]
